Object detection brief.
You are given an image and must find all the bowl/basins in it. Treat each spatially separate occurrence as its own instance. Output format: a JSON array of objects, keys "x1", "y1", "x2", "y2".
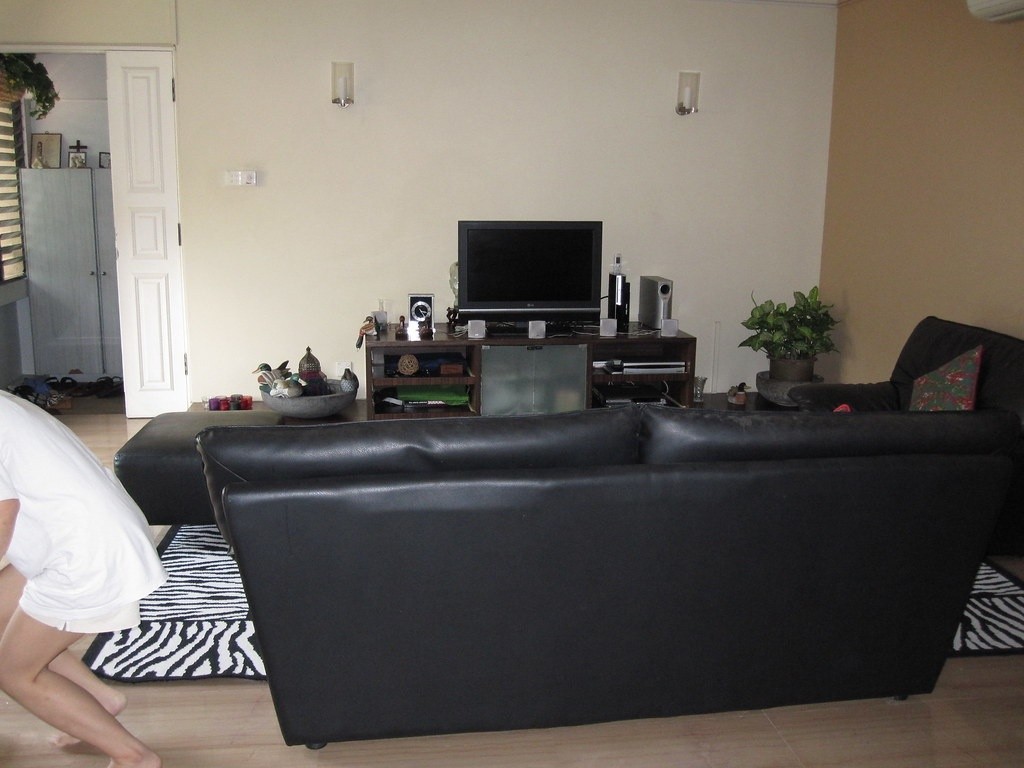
[{"x1": 261, "y1": 379, "x2": 356, "y2": 419}]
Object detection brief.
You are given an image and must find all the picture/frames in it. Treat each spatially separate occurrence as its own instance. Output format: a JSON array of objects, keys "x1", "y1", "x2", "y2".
[
  {"x1": 68, "y1": 152, "x2": 87, "y2": 168},
  {"x1": 30, "y1": 133, "x2": 62, "y2": 169},
  {"x1": 99, "y1": 152, "x2": 110, "y2": 168}
]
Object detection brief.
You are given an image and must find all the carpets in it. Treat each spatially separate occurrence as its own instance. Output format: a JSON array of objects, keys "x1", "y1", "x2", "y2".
[{"x1": 85, "y1": 524, "x2": 1024, "y2": 682}]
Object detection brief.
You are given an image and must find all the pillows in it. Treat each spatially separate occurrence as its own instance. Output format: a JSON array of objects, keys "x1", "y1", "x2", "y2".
[{"x1": 909, "y1": 346, "x2": 982, "y2": 411}]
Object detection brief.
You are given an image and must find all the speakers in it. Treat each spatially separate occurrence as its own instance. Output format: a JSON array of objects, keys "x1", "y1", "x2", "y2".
[
  {"x1": 600, "y1": 319, "x2": 617, "y2": 337},
  {"x1": 468, "y1": 320, "x2": 486, "y2": 338},
  {"x1": 661, "y1": 320, "x2": 679, "y2": 337},
  {"x1": 529, "y1": 321, "x2": 546, "y2": 339}
]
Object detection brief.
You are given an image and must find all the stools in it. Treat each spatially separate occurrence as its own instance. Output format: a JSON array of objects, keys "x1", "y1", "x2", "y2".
[{"x1": 115, "y1": 411, "x2": 283, "y2": 527}]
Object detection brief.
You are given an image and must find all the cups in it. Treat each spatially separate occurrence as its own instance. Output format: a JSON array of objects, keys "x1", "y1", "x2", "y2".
[{"x1": 693, "y1": 376, "x2": 708, "y2": 403}]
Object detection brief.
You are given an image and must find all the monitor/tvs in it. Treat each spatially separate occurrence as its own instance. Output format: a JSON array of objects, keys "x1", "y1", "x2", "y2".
[{"x1": 458, "y1": 220, "x2": 603, "y2": 334}]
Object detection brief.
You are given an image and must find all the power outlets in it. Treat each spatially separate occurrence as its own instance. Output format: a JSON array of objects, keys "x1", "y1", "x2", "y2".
[{"x1": 224, "y1": 171, "x2": 239, "y2": 185}]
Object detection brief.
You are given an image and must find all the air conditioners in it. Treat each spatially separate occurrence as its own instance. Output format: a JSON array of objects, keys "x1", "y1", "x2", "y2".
[{"x1": 966, "y1": 0, "x2": 1024, "y2": 23}]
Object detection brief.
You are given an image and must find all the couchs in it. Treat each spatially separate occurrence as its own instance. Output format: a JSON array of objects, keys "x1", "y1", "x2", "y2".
[
  {"x1": 789, "y1": 315, "x2": 1024, "y2": 414},
  {"x1": 197, "y1": 408, "x2": 1004, "y2": 750}
]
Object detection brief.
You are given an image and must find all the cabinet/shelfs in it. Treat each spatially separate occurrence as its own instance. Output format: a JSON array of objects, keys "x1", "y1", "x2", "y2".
[
  {"x1": 592, "y1": 321, "x2": 696, "y2": 411},
  {"x1": 19, "y1": 168, "x2": 122, "y2": 378},
  {"x1": 364, "y1": 323, "x2": 479, "y2": 420}
]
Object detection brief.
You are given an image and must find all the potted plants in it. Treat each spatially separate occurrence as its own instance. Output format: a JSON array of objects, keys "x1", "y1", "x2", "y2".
[
  {"x1": 0, "y1": 53, "x2": 60, "y2": 121},
  {"x1": 738, "y1": 286, "x2": 842, "y2": 406}
]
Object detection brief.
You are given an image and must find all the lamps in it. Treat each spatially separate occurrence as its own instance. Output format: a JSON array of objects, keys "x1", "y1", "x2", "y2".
[
  {"x1": 676, "y1": 70, "x2": 700, "y2": 116},
  {"x1": 331, "y1": 60, "x2": 354, "y2": 107}
]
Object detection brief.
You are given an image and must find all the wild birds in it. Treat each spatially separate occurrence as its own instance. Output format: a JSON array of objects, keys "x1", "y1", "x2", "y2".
[
  {"x1": 341, "y1": 368, "x2": 359, "y2": 392},
  {"x1": 254, "y1": 363, "x2": 291, "y2": 386},
  {"x1": 259, "y1": 360, "x2": 290, "y2": 385},
  {"x1": 356, "y1": 316, "x2": 377, "y2": 348},
  {"x1": 270, "y1": 378, "x2": 304, "y2": 397}
]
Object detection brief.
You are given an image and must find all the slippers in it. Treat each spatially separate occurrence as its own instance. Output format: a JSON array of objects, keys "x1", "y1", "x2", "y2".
[
  {"x1": 44, "y1": 377, "x2": 59, "y2": 392},
  {"x1": 58, "y1": 376, "x2": 81, "y2": 396},
  {"x1": 82, "y1": 381, "x2": 100, "y2": 397},
  {"x1": 97, "y1": 376, "x2": 123, "y2": 398},
  {"x1": 6, "y1": 384, "x2": 47, "y2": 406}
]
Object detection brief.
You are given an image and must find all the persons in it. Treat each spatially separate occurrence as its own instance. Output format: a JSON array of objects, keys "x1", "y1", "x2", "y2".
[{"x1": 0, "y1": 389, "x2": 172, "y2": 768}]
